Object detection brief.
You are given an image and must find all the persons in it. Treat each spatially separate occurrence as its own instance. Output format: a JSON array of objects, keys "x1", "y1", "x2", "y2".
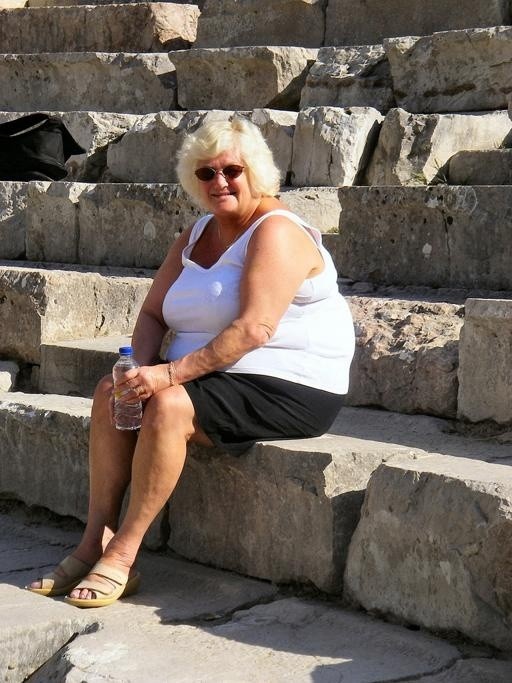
[{"x1": 25, "y1": 118, "x2": 353, "y2": 608}]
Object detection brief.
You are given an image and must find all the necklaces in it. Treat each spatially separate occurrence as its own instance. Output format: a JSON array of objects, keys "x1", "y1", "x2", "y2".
[{"x1": 212, "y1": 214, "x2": 245, "y2": 254}]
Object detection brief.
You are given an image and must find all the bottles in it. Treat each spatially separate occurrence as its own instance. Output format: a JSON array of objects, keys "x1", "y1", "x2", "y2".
[{"x1": 111, "y1": 345, "x2": 145, "y2": 432}]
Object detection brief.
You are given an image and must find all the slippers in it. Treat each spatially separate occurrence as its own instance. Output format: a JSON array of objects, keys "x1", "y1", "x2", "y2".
[
  {"x1": 63, "y1": 561, "x2": 141, "y2": 608},
  {"x1": 25, "y1": 556, "x2": 92, "y2": 597}
]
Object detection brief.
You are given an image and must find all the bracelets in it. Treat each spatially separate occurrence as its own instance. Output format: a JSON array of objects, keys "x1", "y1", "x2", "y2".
[{"x1": 166, "y1": 359, "x2": 178, "y2": 385}]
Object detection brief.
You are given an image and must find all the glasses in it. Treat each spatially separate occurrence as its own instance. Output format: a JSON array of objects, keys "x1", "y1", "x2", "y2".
[{"x1": 192, "y1": 165, "x2": 248, "y2": 181}]
